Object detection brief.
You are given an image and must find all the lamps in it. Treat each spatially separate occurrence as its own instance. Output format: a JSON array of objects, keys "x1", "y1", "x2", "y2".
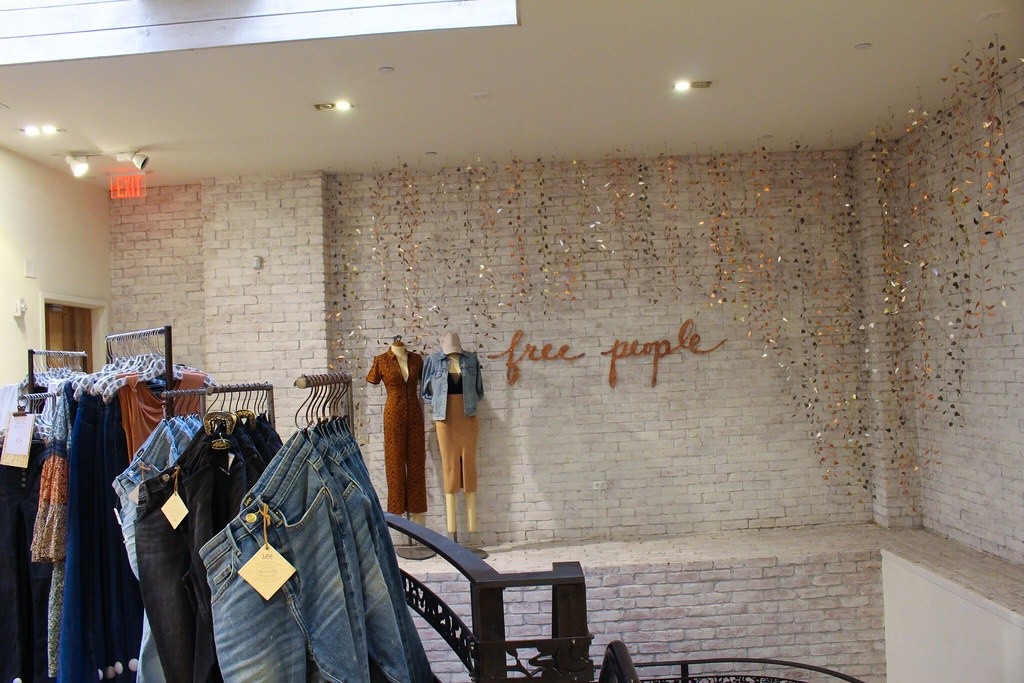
[
  {"x1": 130, "y1": 154, "x2": 149, "y2": 170},
  {"x1": 66, "y1": 157, "x2": 89, "y2": 176}
]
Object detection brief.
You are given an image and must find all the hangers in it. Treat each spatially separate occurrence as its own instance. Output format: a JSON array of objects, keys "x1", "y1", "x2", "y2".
[{"x1": 0, "y1": 326, "x2": 217, "y2": 440}]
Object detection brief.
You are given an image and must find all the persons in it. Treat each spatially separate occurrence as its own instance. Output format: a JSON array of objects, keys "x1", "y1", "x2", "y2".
[
  {"x1": 422, "y1": 331, "x2": 485, "y2": 534},
  {"x1": 366, "y1": 339, "x2": 429, "y2": 514}
]
